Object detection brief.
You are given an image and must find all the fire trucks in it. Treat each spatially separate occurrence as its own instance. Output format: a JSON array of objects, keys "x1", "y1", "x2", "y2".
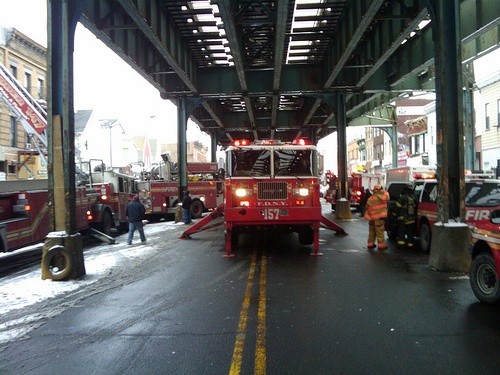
[
  {"x1": 324, "y1": 169, "x2": 384, "y2": 212},
  {"x1": 177, "y1": 137, "x2": 348, "y2": 259},
  {"x1": 0, "y1": 62, "x2": 219, "y2": 255},
  {"x1": 384, "y1": 165, "x2": 500, "y2": 305}
]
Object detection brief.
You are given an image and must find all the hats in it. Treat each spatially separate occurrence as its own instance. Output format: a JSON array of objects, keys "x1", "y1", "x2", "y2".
[{"x1": 183, "y1": 189, "x2": 189, "y2": 194}]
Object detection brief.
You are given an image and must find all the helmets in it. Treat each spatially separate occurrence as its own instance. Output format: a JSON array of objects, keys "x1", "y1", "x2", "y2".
[{"x1": 373, "y1": 185, "x2": 380, "y2": 189}]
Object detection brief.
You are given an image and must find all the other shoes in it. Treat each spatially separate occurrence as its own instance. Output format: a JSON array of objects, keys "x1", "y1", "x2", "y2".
[
  {"x1": 368, "y1": 244, "x2": 376, "y2": 247},
  {"x1": 378, "y1": 245, "x2": 388, "y2": 248}
]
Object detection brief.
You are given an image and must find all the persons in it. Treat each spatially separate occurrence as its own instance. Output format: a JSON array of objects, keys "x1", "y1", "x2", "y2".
[
  {"x1": 365, "y1": 184, "x2": 388, "y2": 249},
  {"x1": 181, "y1": 190, "x2": 193, "y2": 225},
  {"x1": 360, "y1": 186, "x2": 372, "y2": 217},
  {"x1": 391, "y1": 187, "x2": 416, "y2": 249},
  {"x1": 125, "y1": 195, "x2": 147, "y2": 245}
]
